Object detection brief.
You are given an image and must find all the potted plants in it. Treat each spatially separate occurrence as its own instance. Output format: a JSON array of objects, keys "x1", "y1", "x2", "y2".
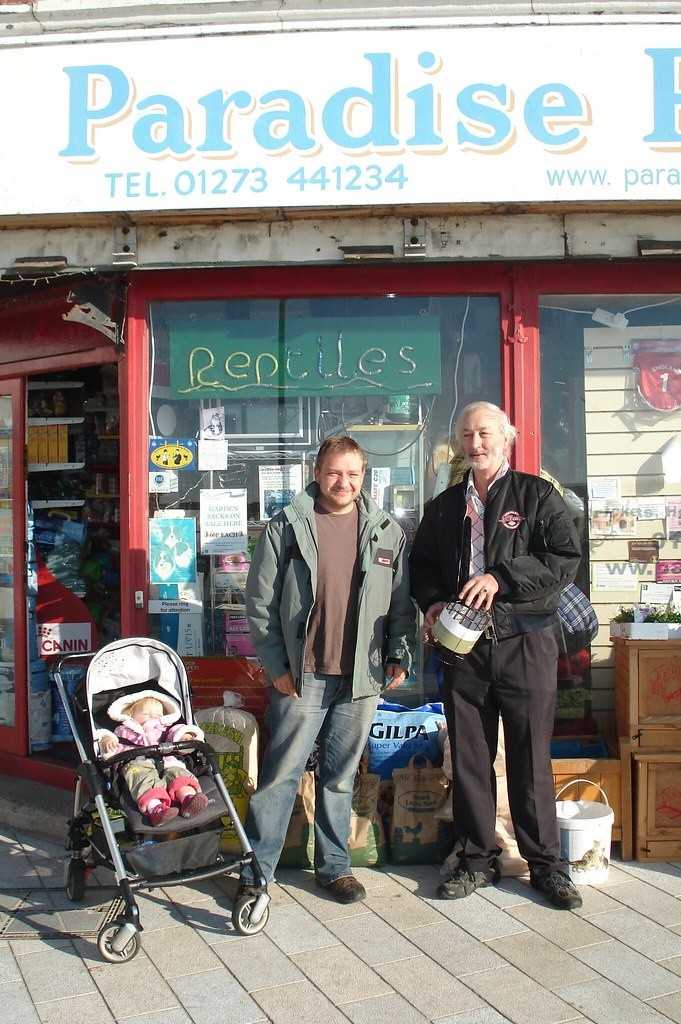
[{"x1": 609, "y1": 606, "x2": 681, "y2": 640}]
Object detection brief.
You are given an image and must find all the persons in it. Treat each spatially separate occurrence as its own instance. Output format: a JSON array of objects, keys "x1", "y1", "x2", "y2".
[
  {"x1": 235, "y1": 435, "x2": 417, "y2": 905},
  {"x1": 96, "y1": 690, "x2": 209, "y2": 828},
  {"x1": 407, "y1": 401, "x2": 584, "y2": 910}
]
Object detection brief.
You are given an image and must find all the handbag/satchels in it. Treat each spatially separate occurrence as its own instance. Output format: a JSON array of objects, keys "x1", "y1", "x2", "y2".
[
  {"x1": 557, "y1": 583, "x2": 598, "y2": 659},
  {"x1": 278, "y1": 771, "x2": 316, "y2": 870},
  {"x1": 348, "y1": 763, "x2": 386, "y2": 867},
  {"x1": 387, "y1": 752, "x2": 451, "y2": 866}
]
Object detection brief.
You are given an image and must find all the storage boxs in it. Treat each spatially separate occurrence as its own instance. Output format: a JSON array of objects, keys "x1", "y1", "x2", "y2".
[
  {"x1": 157, "y1": 585, "x2": 258, "y2": 658},
  {"x1": 551, "y1": 641, "x2": 681, "y2": 864}
]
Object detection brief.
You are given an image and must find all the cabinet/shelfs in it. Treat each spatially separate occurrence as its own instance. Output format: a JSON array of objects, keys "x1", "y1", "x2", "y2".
[{"x1": 24, "y1": 379, "x2": 120, "y2": 527}]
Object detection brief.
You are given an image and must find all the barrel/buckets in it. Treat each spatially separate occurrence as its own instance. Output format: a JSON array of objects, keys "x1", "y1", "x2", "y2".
[{"x1": 554, "y1": 779, "x2": 615, "y2": 886}]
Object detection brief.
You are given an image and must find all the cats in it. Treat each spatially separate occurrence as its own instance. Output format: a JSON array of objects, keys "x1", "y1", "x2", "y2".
[{"x1": 570, "y1": 840, "x2": 609, "y2": 873}]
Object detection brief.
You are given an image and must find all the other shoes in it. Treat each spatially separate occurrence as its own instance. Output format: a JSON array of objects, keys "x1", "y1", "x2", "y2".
[
  {"x1": 149, "y1": 802, "x2": 179, "y2": 827},
  {"x1": 181, "y1": 793, "x2": 208, "y2": 819}
]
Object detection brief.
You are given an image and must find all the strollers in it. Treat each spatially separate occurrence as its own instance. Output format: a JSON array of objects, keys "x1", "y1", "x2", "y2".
[{"x1": 51, "y1": 636, "x2": 272, "y2": 964}]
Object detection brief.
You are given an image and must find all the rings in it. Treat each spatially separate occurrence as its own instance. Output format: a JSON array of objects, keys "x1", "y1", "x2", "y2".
[{"x1": 482, "y1": 588, "x2": 488, "y2": 593}]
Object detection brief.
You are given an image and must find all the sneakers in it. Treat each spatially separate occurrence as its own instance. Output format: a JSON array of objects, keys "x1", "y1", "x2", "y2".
[
  {"x1": 235, "y1": 885, "x2": 253, "y2": 902},
  {"x1": 438, "y1": 866, "x2": 501, "y2": 899},
  {"x1": 530, "y1": 870, "x2": 582, "y2": 910},
  {"x1": 316, "y1": 876, "x2": 366, "y2": 903}
]
